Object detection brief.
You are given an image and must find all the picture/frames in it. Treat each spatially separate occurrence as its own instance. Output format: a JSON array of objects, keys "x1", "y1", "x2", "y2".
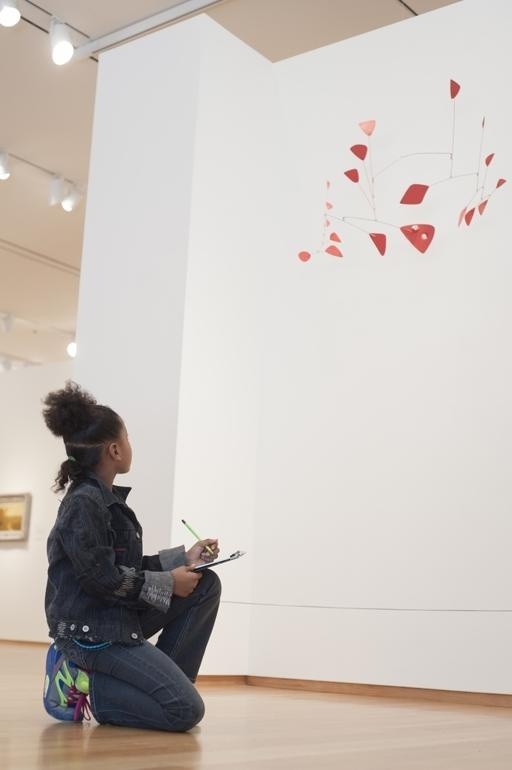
[{"x1": 0, "y1": 493, "x2": 31, "y2": 542}]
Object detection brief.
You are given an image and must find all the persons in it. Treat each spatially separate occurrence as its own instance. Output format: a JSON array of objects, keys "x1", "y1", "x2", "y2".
[{"x1": 42, "y1": 379, "x2": 222, "y2": 732}]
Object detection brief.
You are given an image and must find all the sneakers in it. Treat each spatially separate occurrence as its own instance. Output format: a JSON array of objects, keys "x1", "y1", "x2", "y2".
[{"x1": 43, "y1": 643, "x2": 91, "y2": 721}]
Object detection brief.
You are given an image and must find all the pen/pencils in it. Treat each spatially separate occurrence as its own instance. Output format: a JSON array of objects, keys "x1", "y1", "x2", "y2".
[{"x1": 181, "y1": 519, "x2": 216, "y2": 556}]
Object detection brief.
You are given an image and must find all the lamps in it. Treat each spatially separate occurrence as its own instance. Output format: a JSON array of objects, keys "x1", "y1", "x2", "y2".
[
  {"x1": 0, "y1": 0, "x2": 74, "y2": 66},
  {"x1": 0, "y1": 151, "x2": 85, "y2": 214}
]
